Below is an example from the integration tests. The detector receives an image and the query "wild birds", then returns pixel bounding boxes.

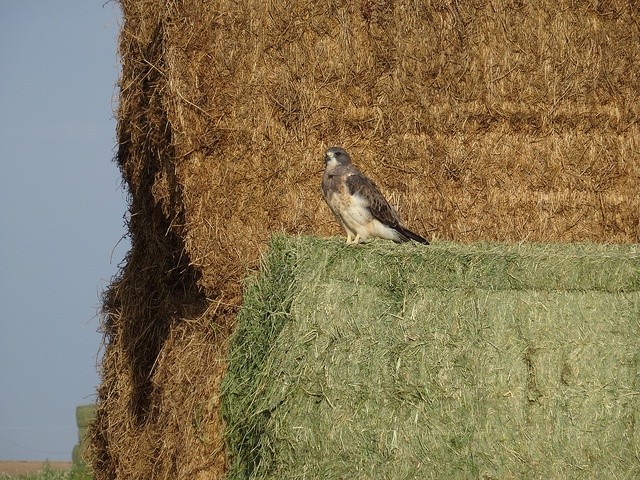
[321,146,429,246]
